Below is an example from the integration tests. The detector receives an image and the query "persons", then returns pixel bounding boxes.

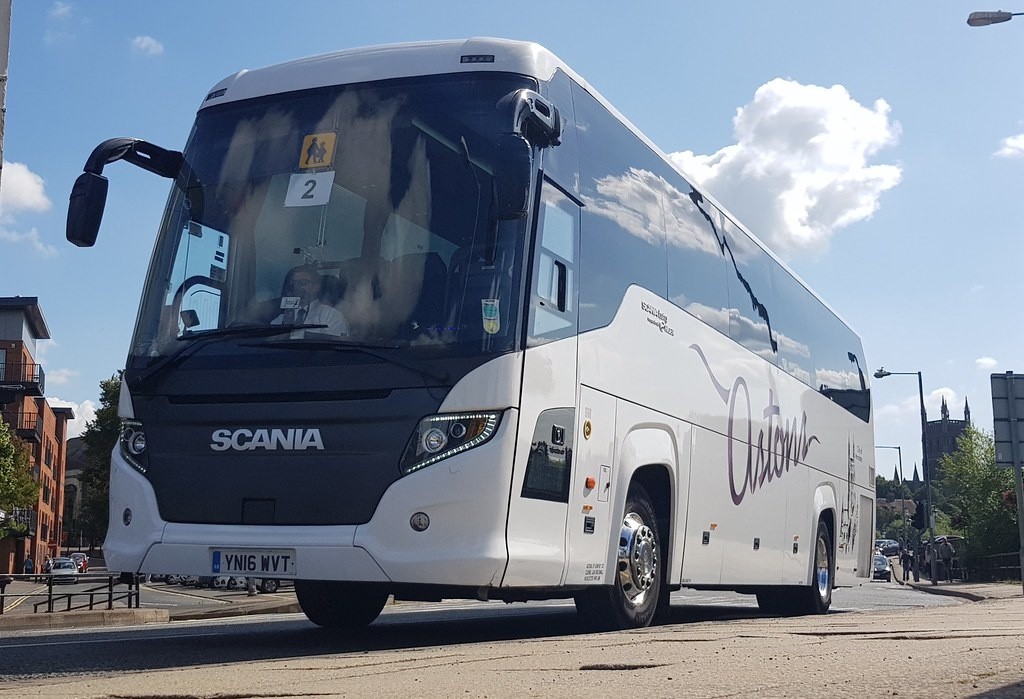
[269,268,349,338]
[43,554,55,581]
[334,257,394,324]
[925,543,937,581]
[82,557,87,573]
[899,549,914,580]
[939,536,955,583]
[24,555,34,580]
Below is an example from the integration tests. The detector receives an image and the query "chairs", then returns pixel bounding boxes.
[319,253,446,303]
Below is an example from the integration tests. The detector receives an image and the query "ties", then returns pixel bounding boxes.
[294,308,306,324]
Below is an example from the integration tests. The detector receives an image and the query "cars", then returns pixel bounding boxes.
[47,559,82,585]
[52,552,91,574]
[875,539,900,556]
[873,554,895,586]
[138,573,294,595]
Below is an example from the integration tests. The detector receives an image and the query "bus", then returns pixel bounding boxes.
[63,35,877,631]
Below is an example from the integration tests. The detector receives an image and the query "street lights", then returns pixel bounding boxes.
[873,364,939,586]
[847,351,866,392]
